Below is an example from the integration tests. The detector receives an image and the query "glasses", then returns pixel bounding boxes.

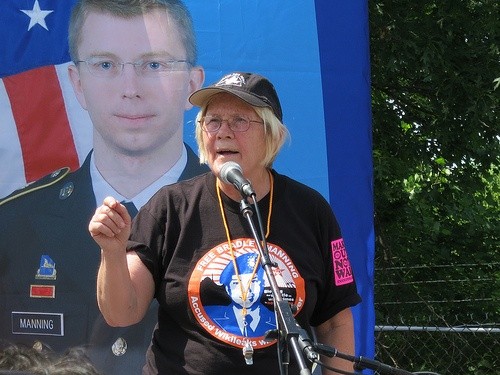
[75,54,193,78]
[196,113,265,133]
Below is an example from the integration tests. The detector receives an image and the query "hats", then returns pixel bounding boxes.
[188,71,283,124]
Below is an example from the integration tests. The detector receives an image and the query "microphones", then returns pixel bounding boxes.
[220,161,256,198]
[293,319,318,363]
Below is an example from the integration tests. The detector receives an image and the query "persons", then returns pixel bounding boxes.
[88,71,363,375]
[0,0,212,375]
[0,337,99,375]
